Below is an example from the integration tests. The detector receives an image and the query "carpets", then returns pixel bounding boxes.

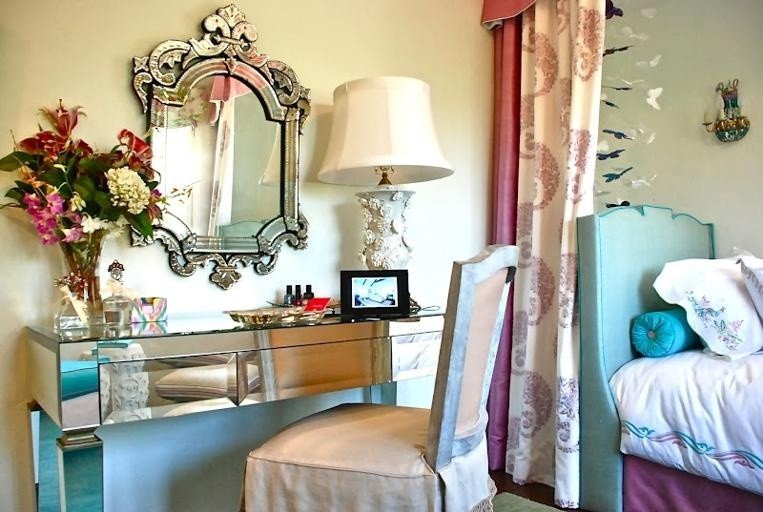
[490,489,567,512]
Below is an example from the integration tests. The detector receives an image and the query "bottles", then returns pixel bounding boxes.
[104,273,133,328]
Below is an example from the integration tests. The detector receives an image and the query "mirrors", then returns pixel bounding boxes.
[133,3,309,290]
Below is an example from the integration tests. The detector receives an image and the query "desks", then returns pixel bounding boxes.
[24,309,445,511]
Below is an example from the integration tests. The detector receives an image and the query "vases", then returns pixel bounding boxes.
[51,230,104,331]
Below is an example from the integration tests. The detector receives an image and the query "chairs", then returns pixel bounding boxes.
[238,242,520,512]
[154,361,262,403]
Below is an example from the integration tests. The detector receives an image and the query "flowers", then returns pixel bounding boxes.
[1,99,193,304]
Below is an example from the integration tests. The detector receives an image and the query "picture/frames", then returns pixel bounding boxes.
[339,270,409,320]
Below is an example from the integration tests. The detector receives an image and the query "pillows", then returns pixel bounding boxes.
[651,247,763,360]
[630,307,697,357]
[737,251,763,321]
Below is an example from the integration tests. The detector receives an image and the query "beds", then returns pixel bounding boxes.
[580,205,763,510]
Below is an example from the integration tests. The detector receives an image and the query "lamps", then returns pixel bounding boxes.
[701,79,750,143]
[318,74,455,309]
